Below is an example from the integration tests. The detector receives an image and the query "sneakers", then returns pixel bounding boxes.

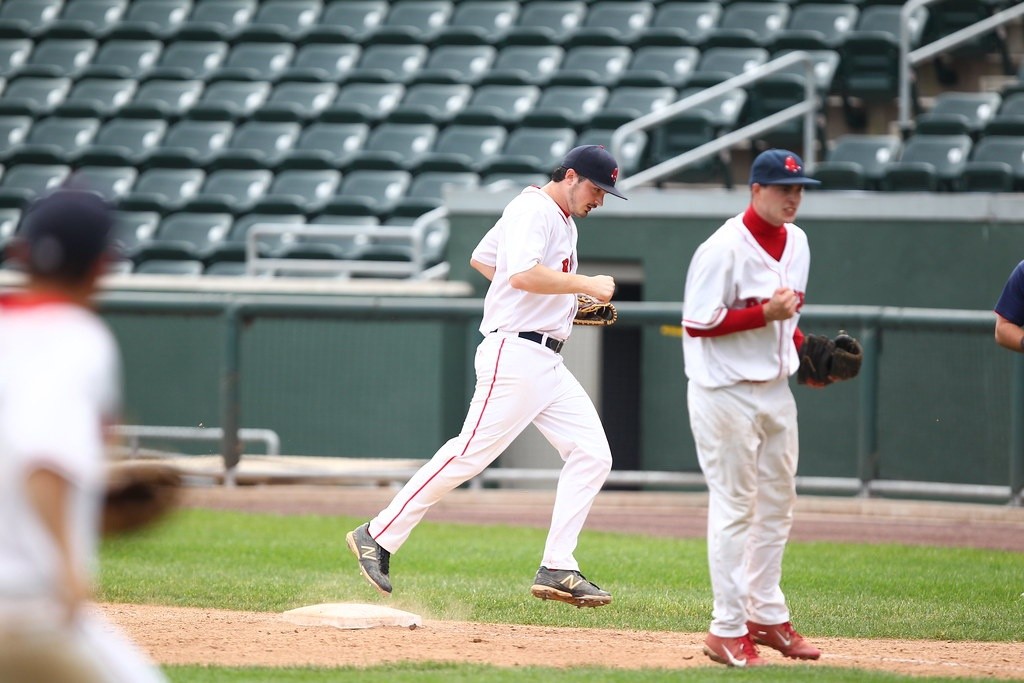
[745,620,821,660]
[345,521,392,593]
[703,632,760,668]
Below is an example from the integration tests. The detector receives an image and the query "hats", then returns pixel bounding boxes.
[749,147,822,187]
[561,144,628,201]
[530,566,611,607]
[0,189,115,282]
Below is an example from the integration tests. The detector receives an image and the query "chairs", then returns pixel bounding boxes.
[0,0,1024,282]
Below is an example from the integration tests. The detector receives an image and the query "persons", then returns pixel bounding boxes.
[0,196,170,683]
[344,146,618,605]
[994,259,1024,352]
[680,149,862,668]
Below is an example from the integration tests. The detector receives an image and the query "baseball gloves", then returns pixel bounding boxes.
[569,292,617,326]
[795,328,864,390]
